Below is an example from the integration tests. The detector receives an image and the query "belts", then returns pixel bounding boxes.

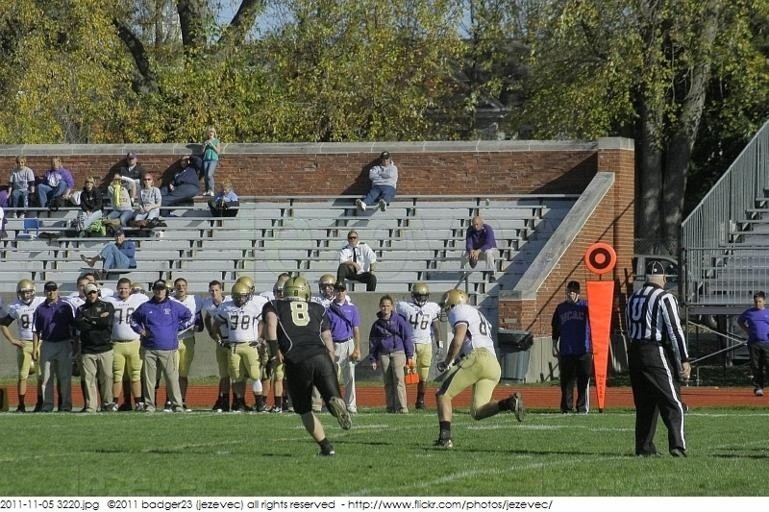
[335,337,353,343]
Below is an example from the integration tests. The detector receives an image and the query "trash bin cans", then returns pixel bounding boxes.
[497,329,532,385]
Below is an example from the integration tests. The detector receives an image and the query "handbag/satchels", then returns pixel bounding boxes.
[84,216,167,237]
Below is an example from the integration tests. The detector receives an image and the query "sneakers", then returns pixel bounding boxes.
[80,254,95,267]
[331,397,352,431]
[669,446,689,458]
[356,198,388,212]
[510,392,525,422]
[317,449,334,456]
[638,453,663,458]
[431,439,453,451]
[16,403,427,415]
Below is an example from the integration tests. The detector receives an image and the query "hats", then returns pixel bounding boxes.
[44,282,57,291]
[127,151,135,157]
[334,280,345,291]
[153,280,167,291]
[646,260,667,275]
[84,283,98,294]
[381,150,389,159]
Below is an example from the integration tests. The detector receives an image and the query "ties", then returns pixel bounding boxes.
[353,248,356,263]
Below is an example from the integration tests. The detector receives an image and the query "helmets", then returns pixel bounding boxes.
[132,283,145,292]
[232,283,251,296]
[438,289,470,307]
[273,278,287,290]
[165,278,175,290]
[16,279,36,296]
[284,276,312,302]
[318,274,336,286]
[567,280,580,292]
[237,276,255,292]
[411,282,431,295]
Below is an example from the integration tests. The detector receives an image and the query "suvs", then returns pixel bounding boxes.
[632,253,718,330]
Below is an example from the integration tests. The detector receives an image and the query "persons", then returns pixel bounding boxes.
[355,151,398,211]
[428,288,526,450]
[0,126,362,456]
[335,230,377,293]
[463,215,500,272]
[737,290,769,396]
[368,295,416,416]
[624,260,691,459]
[550,281,593,414]
[395,283,444,410]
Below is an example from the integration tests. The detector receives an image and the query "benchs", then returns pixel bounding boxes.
[696,188,769,306]
